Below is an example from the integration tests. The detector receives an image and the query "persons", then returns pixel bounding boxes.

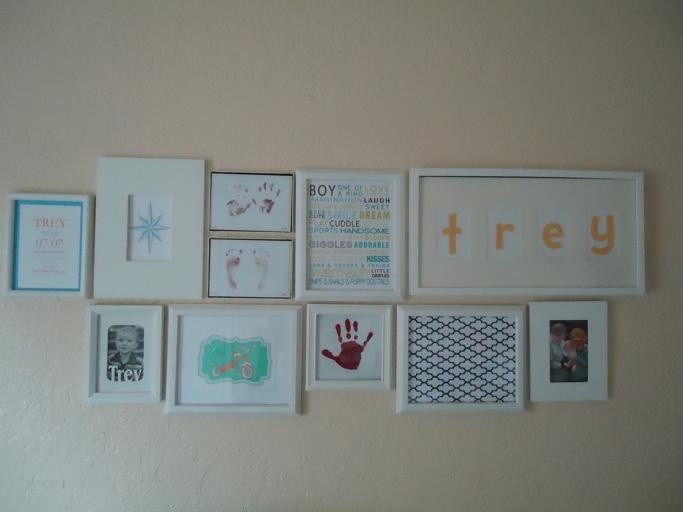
[107,325,143,382]
[549,322,586,383]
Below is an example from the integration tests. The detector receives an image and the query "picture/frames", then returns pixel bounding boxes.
[92,155,205,300]
[396,302,527,413]
[526,300,608,403]
[206,237,293,300]
[207,169,293,234]
[294,168,407,304]
[305,304,394,391]
[81,303,164,404]
[408,166,646,298]
[2,190,94,300]
[164,304,304,418]
